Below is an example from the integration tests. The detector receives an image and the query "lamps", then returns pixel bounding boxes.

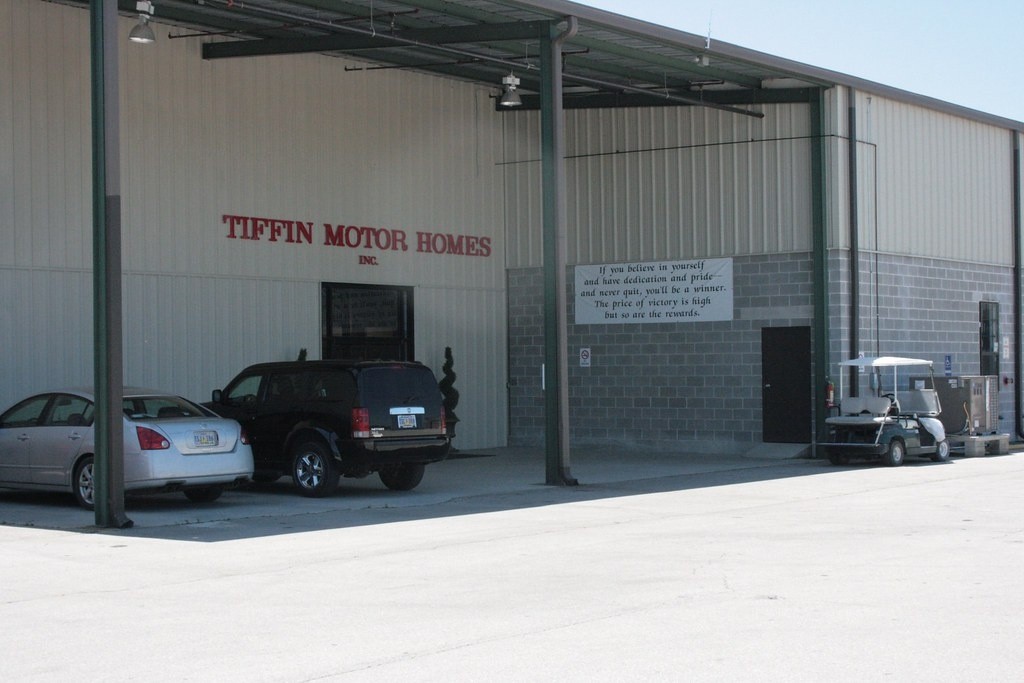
[128,0,156,43]
[500,69,522,107]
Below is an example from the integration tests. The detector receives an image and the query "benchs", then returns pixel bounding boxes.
[825,397,893,424]
[889,398,918,429]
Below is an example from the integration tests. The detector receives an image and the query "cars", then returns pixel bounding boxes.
[0,389,256,509]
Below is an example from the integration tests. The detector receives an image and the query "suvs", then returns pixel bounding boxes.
[158,360,451,501]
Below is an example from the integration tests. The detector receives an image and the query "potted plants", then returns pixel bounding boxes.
[438,346,461,438]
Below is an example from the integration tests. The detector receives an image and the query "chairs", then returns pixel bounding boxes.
[158,406,178,417]
[124,407,134,418]
[68,414,86,426]
